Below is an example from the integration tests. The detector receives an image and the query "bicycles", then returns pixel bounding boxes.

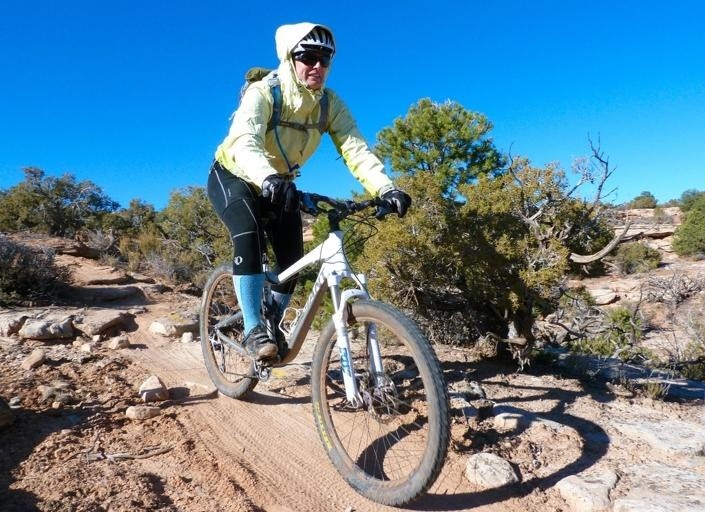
[197,187,452,506]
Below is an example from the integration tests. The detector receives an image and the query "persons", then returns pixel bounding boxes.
[206,20,412,360]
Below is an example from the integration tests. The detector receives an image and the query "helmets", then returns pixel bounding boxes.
[291,28,335,58]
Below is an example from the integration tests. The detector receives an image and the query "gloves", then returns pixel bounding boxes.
[374,188,411,219]
[263,172,301,214]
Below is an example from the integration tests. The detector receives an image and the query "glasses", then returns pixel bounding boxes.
[293,53,329,68]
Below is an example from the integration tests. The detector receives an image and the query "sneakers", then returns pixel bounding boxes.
[241,324,280,361]
